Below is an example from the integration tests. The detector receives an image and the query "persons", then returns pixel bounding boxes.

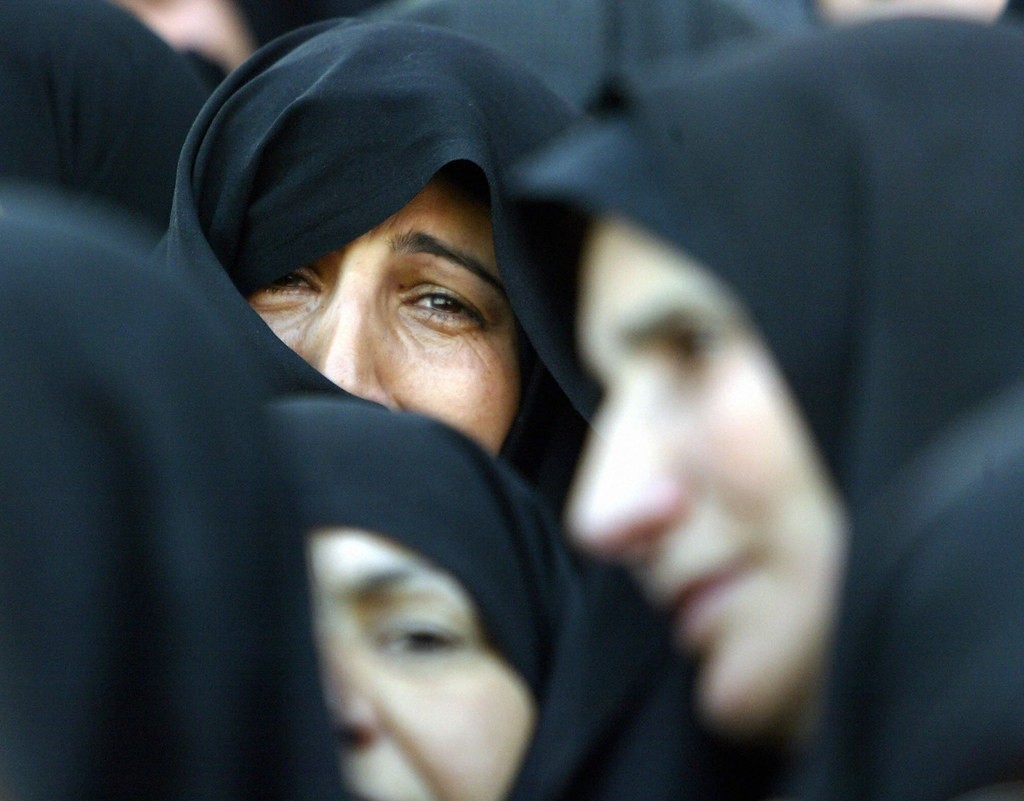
[277,400,565,801]
[167,20,591,516]
[505,18,1024,801]
